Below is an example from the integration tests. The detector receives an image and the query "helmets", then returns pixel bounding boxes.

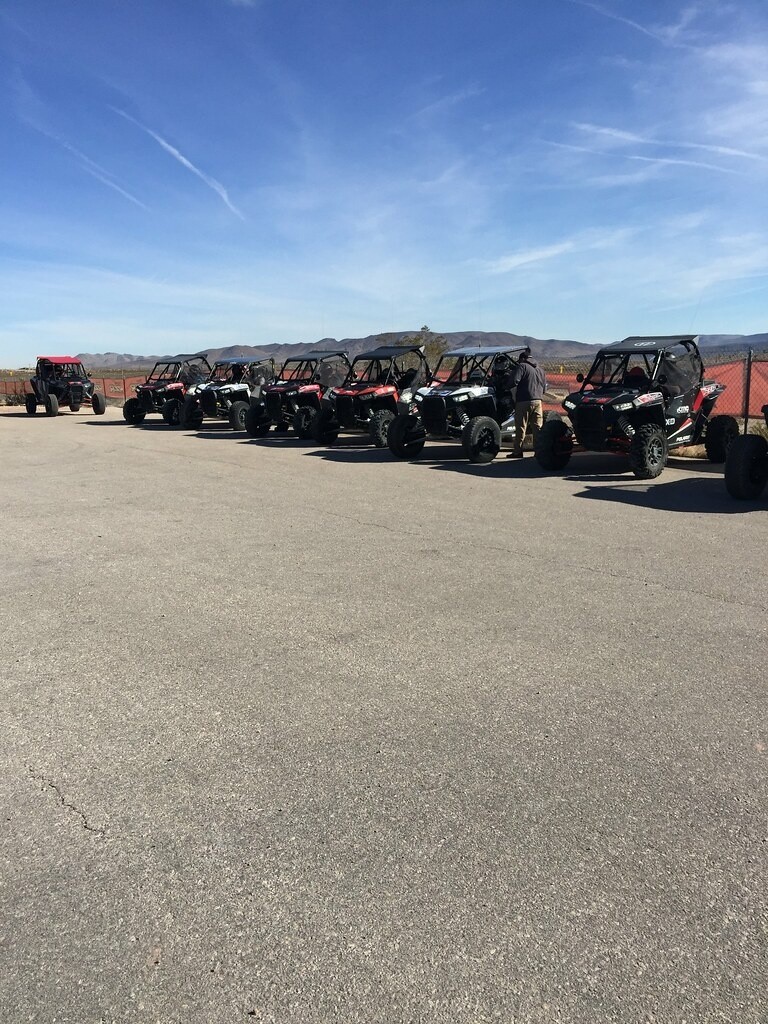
[652,352,676,362]
[493,356,509,370]
[469,369,482,380]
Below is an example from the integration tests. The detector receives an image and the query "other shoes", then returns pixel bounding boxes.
[507,453,523,458]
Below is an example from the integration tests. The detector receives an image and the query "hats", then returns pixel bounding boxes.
[515,351,530,362]
[628,367,644,375]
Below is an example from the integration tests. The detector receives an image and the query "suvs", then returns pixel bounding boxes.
[25,355,106,417]
[532,334,742,480]
[177,355,280,431]
[243,348,362,438]
[723,401,768,502]
[386,344,562,465]
[122,351,220,426]
[307,343,441,447]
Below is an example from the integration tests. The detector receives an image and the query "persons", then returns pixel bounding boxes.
[490,356,516,418]
[55,364,68,378]
[231,365,249,386]
[318,363,342,389]
[374,366,416,391]
[625,367,648,388]
[245,364,264,386]
[186,365,204,384]
[505,352,546,459]
[653,352,690,394]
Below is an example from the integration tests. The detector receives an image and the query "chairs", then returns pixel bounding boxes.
[190,364,205,382]
[396,368,418,389]
[624,367,650,385]
[171,370,188,383]
[376,368,398,382]
[231,367,248,383]
[470,369,482,382]
